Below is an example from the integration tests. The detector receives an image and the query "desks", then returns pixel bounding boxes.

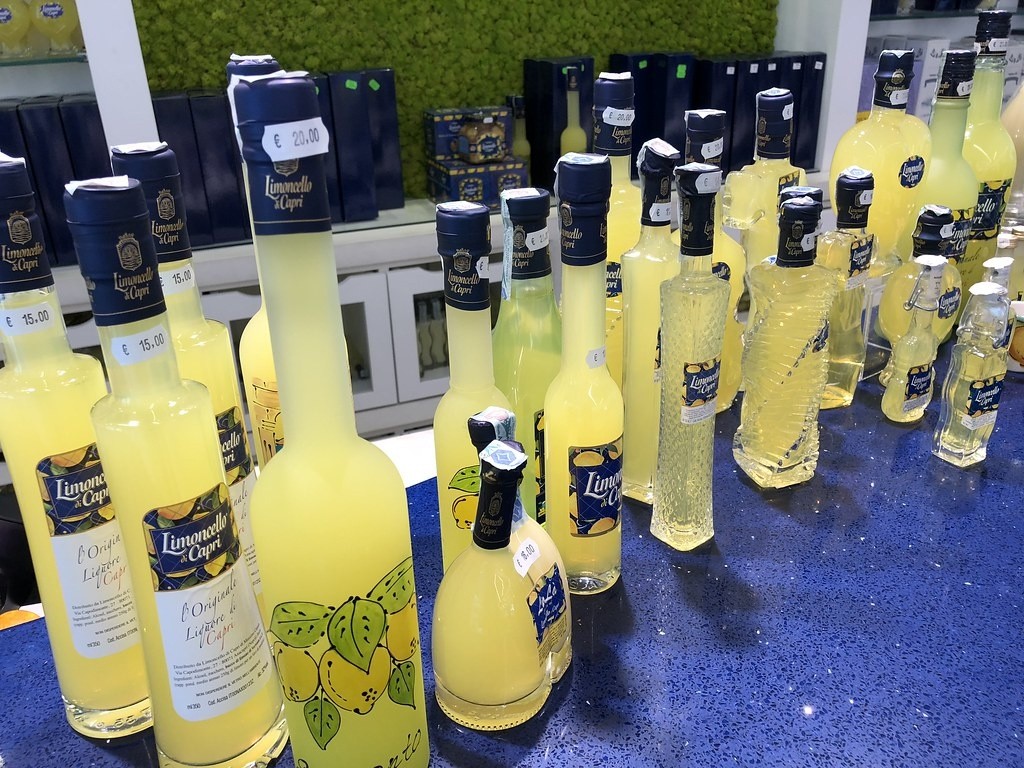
[0,304,1024,768]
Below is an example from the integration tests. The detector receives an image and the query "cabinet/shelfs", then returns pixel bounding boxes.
[773,0,1024,210]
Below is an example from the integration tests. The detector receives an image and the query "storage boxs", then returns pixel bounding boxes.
[362,66,405,210]
[188,89,247,245]
[524,56,580,197]
[609,52,653,180]
[858,33,1024,126]
[470,106,514,156]
[308,72,343,224]
[578,55,595,153]
[691,51,826,180]
[440,164,489,207]
[423,157,457,204]
[152,92,214,248]
[0,95,58,268]
[421,107,475,162]
[320,70,380,222]
[489,159,528,212]
[18,95,78,267]
[648,52,698,181]
[59,93,113,180]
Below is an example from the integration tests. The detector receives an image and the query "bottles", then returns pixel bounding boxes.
[62,176,288,768]
[232,73,431,768]
[0,157,153,739]
[226,49,290,471]
[432,0,1024,732]
[98,141,259,602]
